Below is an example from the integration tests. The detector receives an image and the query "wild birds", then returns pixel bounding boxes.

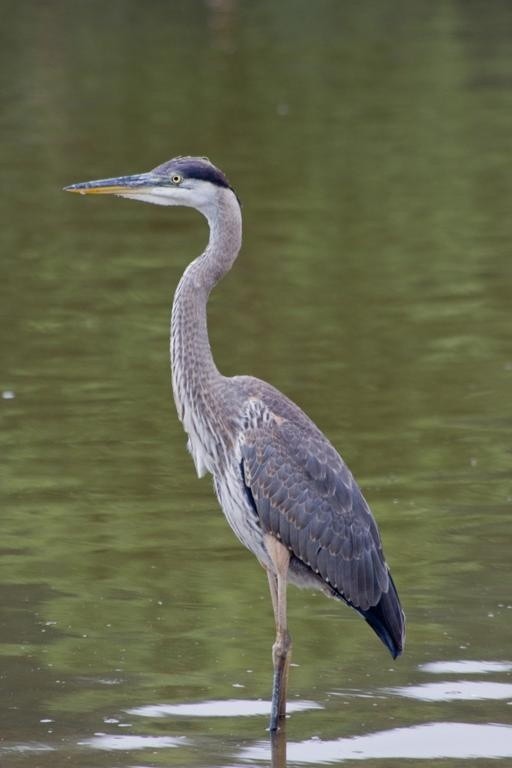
[60,153,406,736]
